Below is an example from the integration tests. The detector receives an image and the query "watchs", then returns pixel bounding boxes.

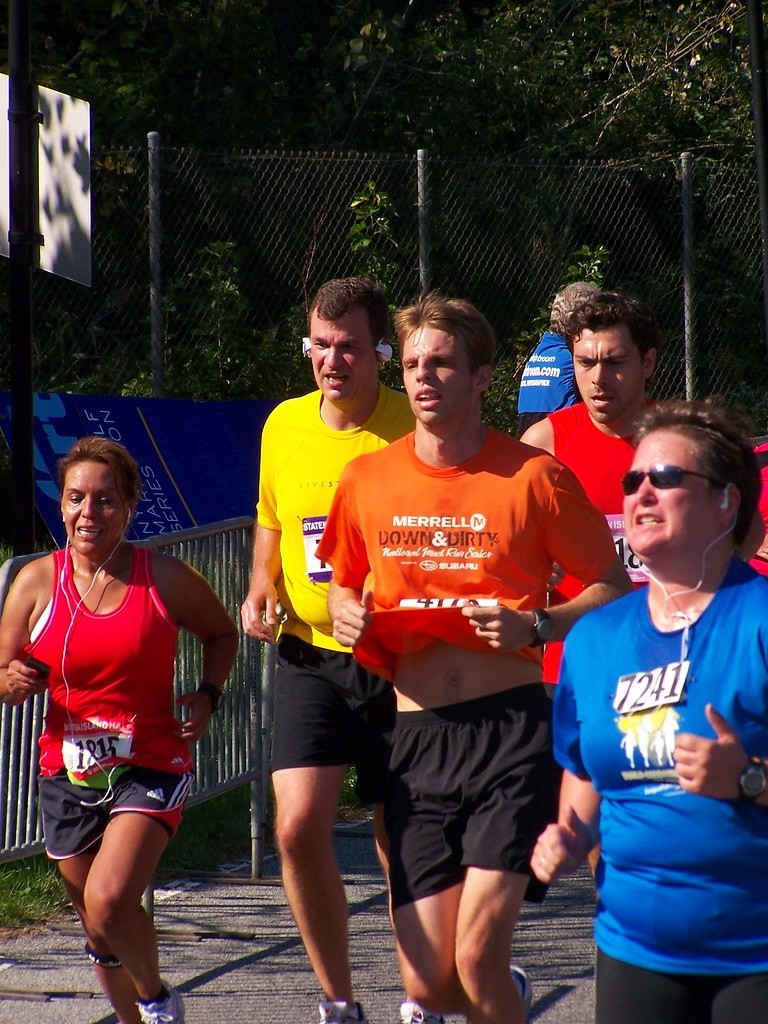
[530,608,556,649]
[736,752,767,806]
[197,681,223,715]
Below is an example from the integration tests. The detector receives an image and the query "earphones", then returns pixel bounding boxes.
[720,488,729,510]
[376,337,392,362]
[63,516,65,522]
[128,508,131,523]
[302,337,311,358]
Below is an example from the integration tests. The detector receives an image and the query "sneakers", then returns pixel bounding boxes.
[318,1001,368,1024]
[400,1002,444,1024]
[509,965,530,1023]
[136,979,184,1024]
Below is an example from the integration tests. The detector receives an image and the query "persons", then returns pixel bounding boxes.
[515,282,599,435]
[0,435,241,1024]
[315,291,631,1024]
[526,398,768,1024]
[520,294,668,874]
[242,282,534,1023]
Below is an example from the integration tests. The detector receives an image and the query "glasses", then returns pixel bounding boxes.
[620,466,725,495]
